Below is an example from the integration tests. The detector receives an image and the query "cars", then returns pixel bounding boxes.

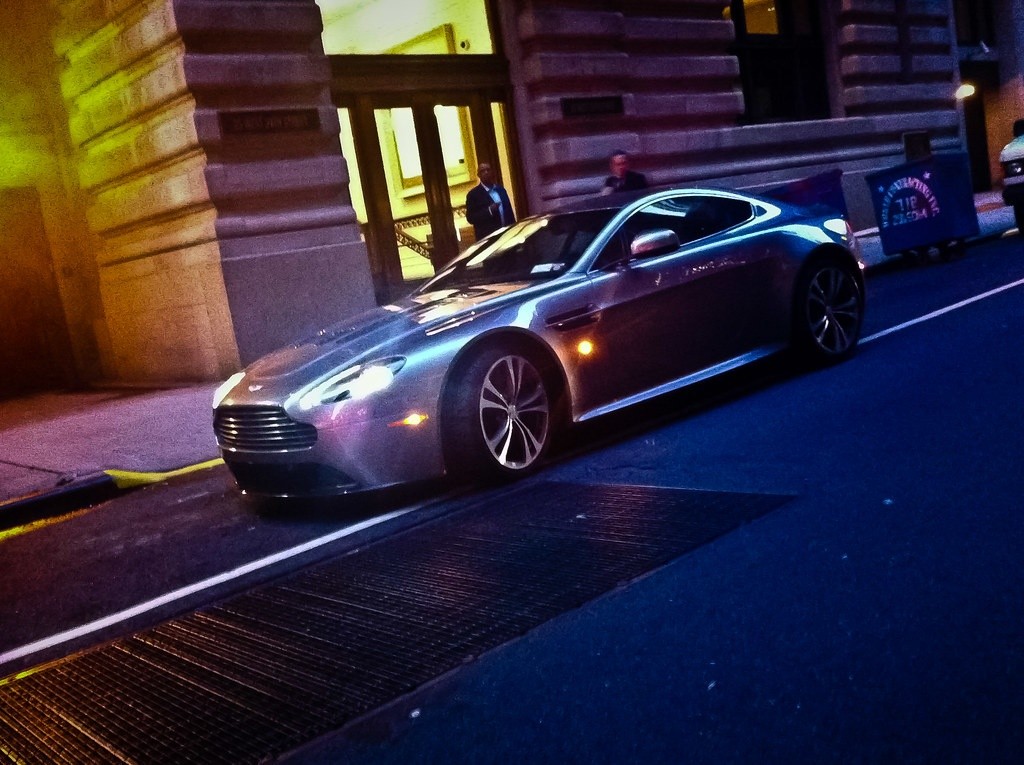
[208,185,869,505]
[998,133,1024,236]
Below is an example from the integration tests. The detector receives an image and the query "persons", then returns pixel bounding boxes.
[999,117,1024,238]
[602,151,646,195]
[466,163,516,242]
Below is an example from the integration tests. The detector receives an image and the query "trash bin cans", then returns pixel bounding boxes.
[863,150,982,256]
[757,168,850,221]
